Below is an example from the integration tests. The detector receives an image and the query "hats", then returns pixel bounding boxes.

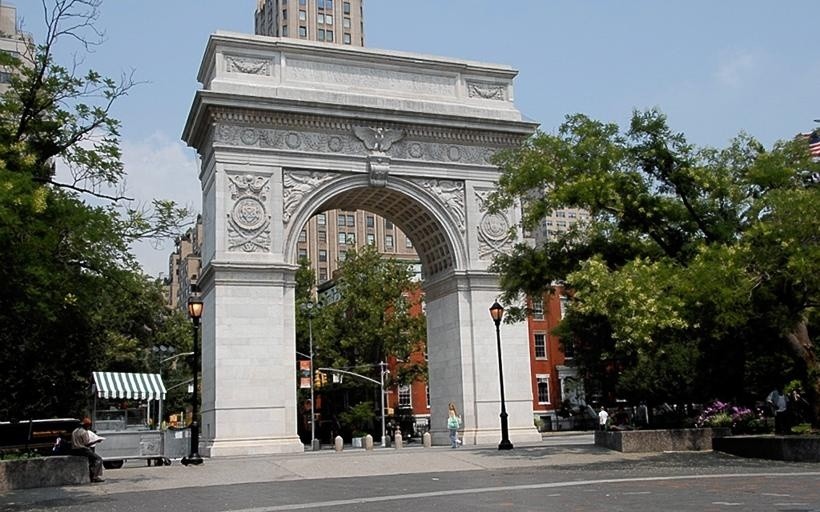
[78,417,93,426]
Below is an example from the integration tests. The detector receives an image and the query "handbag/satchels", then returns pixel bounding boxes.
[446,410,464,430]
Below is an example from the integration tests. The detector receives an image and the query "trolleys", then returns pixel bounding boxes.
[86,371,173,467]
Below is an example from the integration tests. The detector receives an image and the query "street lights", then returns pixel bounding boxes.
[185,293,204,465]
[490,297,515,450]
[152,344,175,374]
[305,300,323,451]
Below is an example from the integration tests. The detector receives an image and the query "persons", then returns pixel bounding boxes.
[447,403,461,448]
[765,383,789,430]
[598,406,608,432]
[70,417,105,483]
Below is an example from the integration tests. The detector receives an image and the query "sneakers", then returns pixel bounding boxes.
[91,476,105,482]
